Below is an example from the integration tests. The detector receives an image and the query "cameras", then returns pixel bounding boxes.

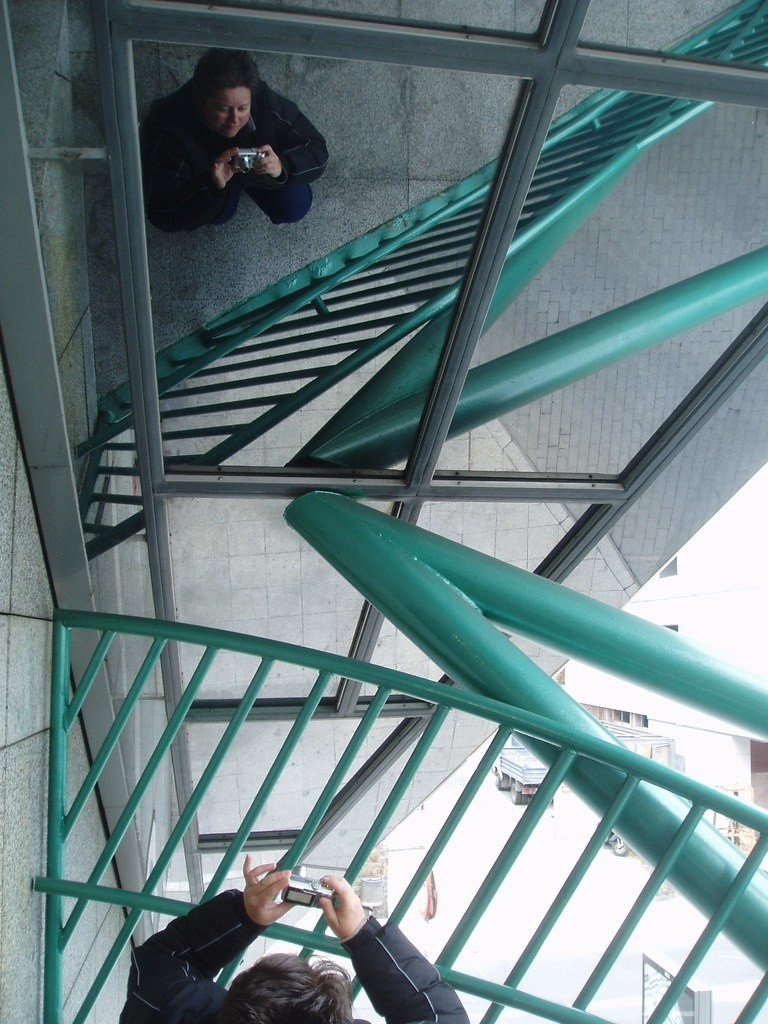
[231,147,266,174]
[281,875,334,909]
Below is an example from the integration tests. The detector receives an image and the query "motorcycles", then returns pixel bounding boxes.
[605,831,629,857]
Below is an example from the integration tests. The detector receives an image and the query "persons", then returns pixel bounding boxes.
[118,853,467,1024]
[141,47,328,233]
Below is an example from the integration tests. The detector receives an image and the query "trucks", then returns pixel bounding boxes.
[492,747,554,806]
